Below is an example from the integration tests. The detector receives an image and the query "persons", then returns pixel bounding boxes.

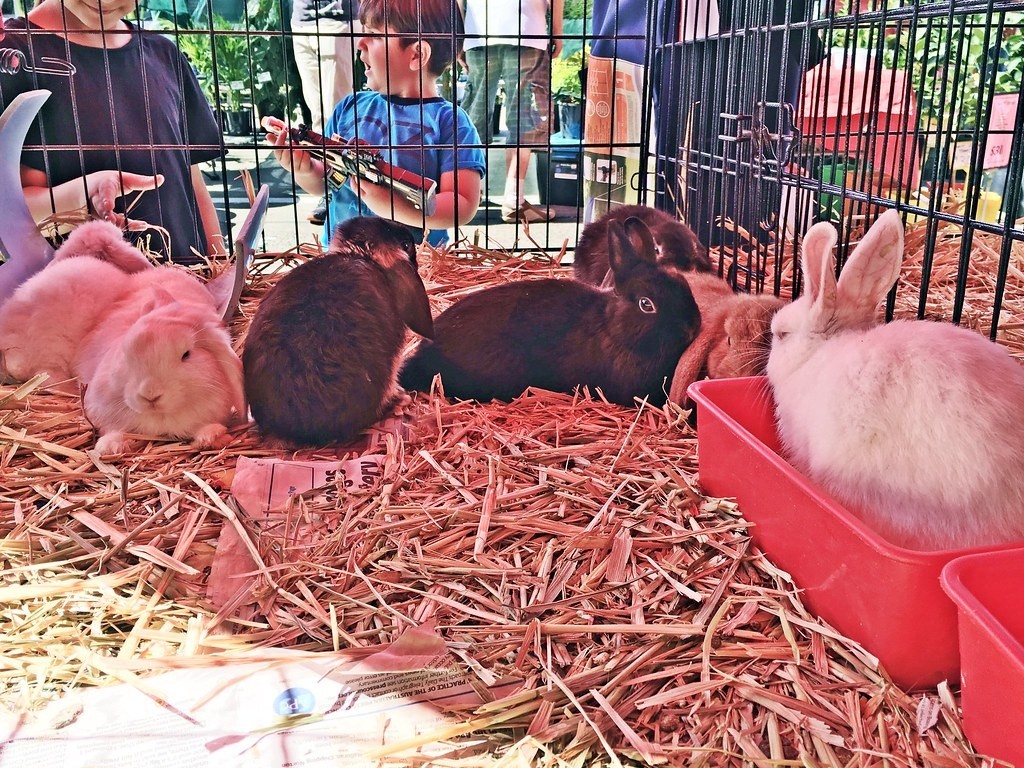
[645,0,828,249]
[456,0,562,223]
[0,0,231,270]
[265,0,487,255]
[290,0,363,223]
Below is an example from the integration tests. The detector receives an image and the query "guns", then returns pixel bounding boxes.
[259,115,439,218]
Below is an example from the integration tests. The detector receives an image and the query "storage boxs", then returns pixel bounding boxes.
[537,131,584,208]
[687,376,1024,691]
[940,546,1024,768]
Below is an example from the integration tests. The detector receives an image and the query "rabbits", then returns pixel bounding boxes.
[394,215,703,409]
[0,217,155,389]
[71,263,248,457]
[667,266,786,408]
[241,216,437,447]
[571,203,714,290]
[764,205,1024,552]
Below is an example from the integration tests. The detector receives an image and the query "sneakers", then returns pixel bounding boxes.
[306,194,332,222]
[502,200,556,222]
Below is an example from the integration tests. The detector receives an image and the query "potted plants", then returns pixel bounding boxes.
[174,11,287,135]
[558,69,583,140]
